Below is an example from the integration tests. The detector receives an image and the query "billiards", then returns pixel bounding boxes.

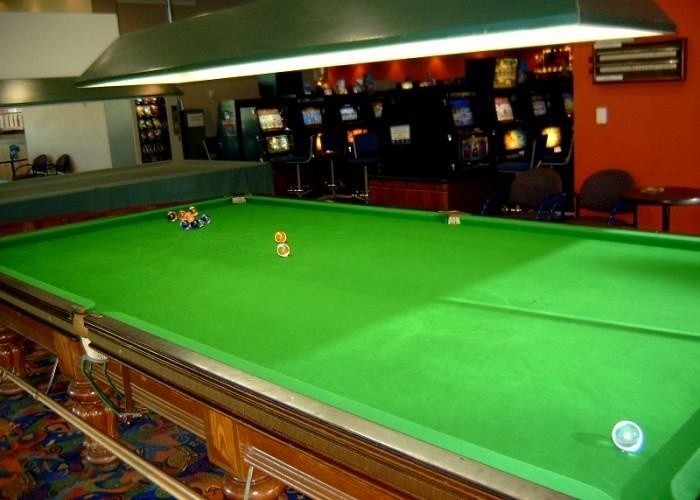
[167,211,177,222]
[178,206,211,231]
[275,232,286,243]
[612,420,644,454]
[277,245,291,259]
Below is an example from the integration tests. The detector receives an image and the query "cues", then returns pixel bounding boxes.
[252,446,354,498]
[432,296,699,340]
[246,453,329,498]
[248,448,343,498]
[243,457,317,498]
[1,368,202,500]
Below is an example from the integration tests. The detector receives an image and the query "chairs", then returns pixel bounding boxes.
[500,140,537,176]
[39,154,69,177]
[578,168,638,231]
[541,127,574,169]
[13,155,46,180]
[202,137,226,160]
[284,132,378,203]
[479,168,566,223]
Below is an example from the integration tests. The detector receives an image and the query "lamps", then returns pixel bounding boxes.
[592,36,686,85]
[74,1,678,91]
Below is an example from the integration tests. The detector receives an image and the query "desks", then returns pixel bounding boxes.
[370,172,485,211]
[625,186,700,232]
[2,161,275,238]
[1,195,700,500]
[0,157,27,182]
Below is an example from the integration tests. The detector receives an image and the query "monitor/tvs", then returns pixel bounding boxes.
[256,103,411,154]
[448,93,574,161]
